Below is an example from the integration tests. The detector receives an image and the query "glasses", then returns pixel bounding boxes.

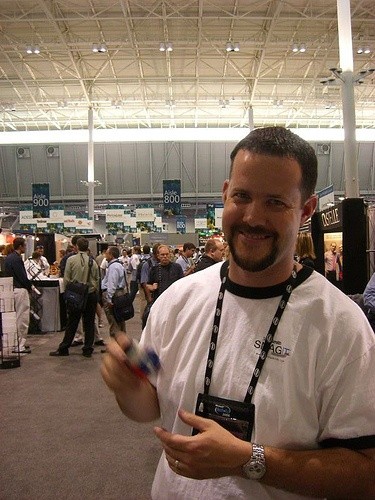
[36,245,44,250]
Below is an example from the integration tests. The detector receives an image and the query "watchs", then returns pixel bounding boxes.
[240,443,266,481]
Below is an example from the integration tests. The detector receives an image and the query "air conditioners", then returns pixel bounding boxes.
[45,145,59,157]
[316,144,330,155]
[16,146,31,159]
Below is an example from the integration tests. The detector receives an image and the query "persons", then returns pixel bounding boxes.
[363,272,375,310]
[118,242,184,305]
[24,245,50,280]
[324,242,343,282]
[49,235,128,358]
[4,237,36,354]
[0,244,15,257]
[295,232,316,268]
[168,238,225,276]
[100,126,374,500]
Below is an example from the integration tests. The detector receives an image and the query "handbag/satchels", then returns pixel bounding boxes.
[110,293,134,324]
[64,279,89,309]
[142,304,150,330]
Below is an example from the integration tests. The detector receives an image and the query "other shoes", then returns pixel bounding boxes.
[70,341,83,346]
[49,349,70,356]
[82,351,91,357]
[24,345,30,349]
[20,350,31,354]
[30,329,49,334]
[94,340,104,346]
[98,318,104,328]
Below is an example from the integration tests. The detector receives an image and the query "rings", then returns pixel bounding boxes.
[175,460,179,467]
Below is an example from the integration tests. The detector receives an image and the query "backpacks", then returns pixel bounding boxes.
[137,258,149,280]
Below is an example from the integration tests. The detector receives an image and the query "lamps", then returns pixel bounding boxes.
[90,42,107,53]
[160,42,174,52]
[26,44,41,55]
[355,46,371,54]
[293,43,306,52]
[226,42,241,53]
